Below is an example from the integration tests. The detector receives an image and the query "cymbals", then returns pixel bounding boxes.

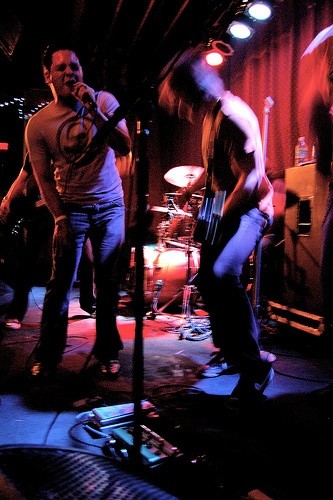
[163,165,207,188]
[164,192,204,199]
[150,206,194,217]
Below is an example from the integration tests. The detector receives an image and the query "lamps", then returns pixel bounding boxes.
[204,0,285,66]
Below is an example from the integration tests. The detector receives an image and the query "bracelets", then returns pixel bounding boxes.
[189,185,195,190]
[54,215,68,224]
[3,196,8,199]
[90,108,99,113]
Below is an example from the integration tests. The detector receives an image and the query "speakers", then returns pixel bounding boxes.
[280,161,331,328]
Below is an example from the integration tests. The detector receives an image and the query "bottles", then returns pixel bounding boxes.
[294,136,308,166]
[312,138,318,160]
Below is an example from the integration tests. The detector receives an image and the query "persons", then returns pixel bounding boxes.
[160,58,274,400]
[0,44,136,385]
[309,72,333,334]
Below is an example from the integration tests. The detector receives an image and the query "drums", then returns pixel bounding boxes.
[136,241,201,309]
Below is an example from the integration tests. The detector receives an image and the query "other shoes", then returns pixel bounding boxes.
[29,354,51,380]
[2,317,22,330]
[95,357,122,379]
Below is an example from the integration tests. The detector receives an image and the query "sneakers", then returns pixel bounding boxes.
[198,356,237,378]
[227,362,275,411]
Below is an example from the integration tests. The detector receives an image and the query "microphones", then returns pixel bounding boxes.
[69,82,96,107]
